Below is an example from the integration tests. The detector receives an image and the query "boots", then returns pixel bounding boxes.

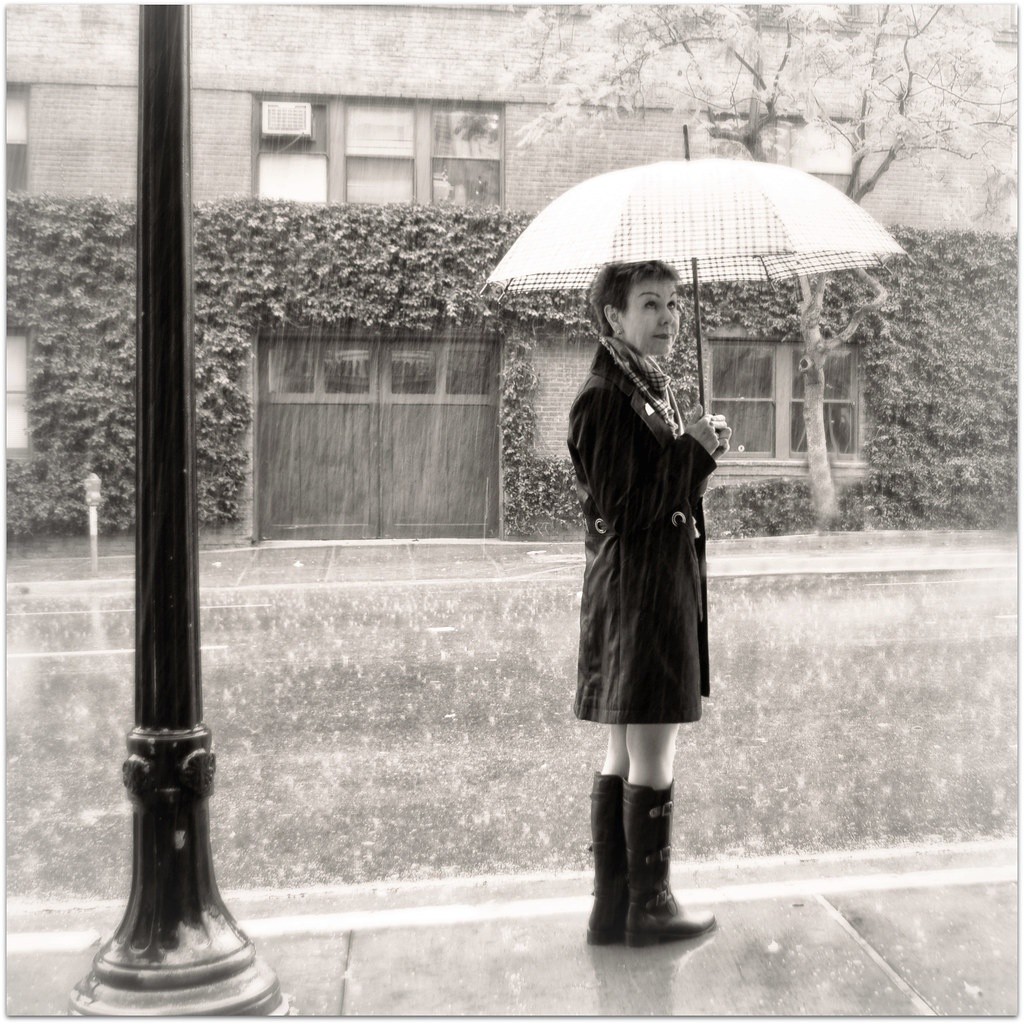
[623,778,717,947]
[587,771,628,944]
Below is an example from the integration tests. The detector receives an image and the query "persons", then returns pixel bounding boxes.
[565,261,732,947]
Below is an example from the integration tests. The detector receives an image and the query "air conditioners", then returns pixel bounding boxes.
[262,99,311,141]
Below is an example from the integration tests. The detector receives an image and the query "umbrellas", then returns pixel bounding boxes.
[477,126,911,414]
